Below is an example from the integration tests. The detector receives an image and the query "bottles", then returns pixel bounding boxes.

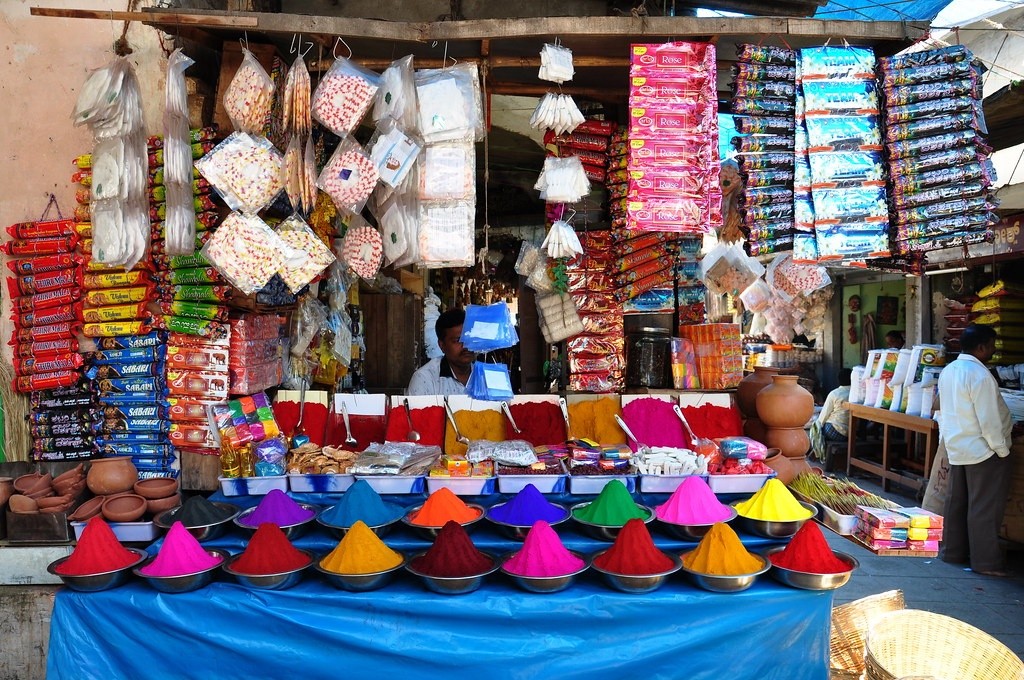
[625,327,672,388]
[737,367,822,501]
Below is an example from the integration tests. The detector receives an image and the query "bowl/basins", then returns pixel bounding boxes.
[0,457,861,591]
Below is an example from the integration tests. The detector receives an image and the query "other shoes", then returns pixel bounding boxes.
[980,565,1019,577]
[943,553,971,563]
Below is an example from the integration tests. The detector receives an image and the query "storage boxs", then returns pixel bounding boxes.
[216,456,779,494]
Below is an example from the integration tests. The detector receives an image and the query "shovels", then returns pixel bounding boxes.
[295,378,307,436]
[673,404,722,452]
[403,397,421,441]
[613,414,651,453]
[342,401,358,448]
[443,396,474,447]
[500,400,522,434]
[559,397,582,441]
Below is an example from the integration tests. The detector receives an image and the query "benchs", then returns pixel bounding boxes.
[824,434,905,471]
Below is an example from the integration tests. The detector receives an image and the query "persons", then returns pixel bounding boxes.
[407,307,474,397]
[938,325,1014,577]
[809,330,907,462]
